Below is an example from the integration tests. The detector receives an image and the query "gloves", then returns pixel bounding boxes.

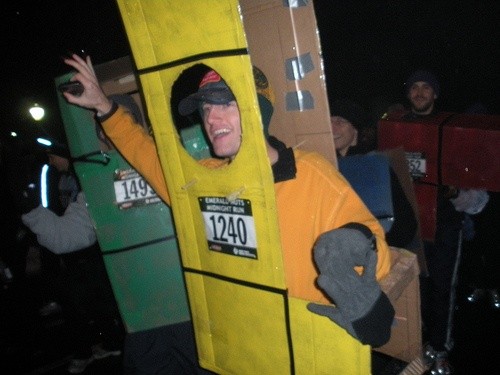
[305,222,397,347]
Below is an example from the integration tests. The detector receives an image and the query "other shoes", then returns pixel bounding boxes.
[66,343,121,373]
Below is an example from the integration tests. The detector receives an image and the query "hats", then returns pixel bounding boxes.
[402,71,440,91]
[329,95,361,130]
[178,61,274,118]
